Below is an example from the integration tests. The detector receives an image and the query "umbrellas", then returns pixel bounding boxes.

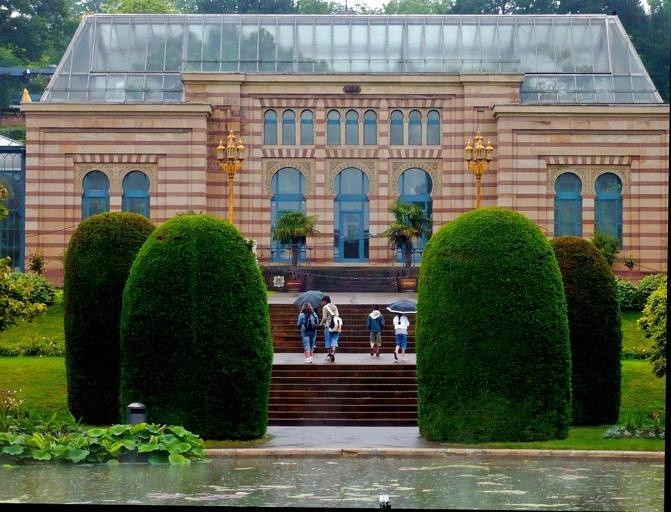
[386,300,418,313]
[293,290,325,309]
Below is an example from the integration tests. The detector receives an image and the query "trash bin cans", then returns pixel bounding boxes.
[128,402,147,424]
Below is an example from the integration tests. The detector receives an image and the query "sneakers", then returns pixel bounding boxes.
[370,348,379,356]
[305,357,313,364]
[325,353,336,364]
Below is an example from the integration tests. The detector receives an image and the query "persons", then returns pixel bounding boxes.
[297,296,343,364]
[367,305,410,360]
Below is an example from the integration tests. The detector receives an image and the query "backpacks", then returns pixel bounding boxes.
[323,305,343,334]
[303,310,319,330]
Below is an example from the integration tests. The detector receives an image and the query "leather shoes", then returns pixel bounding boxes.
[393,351,407,361]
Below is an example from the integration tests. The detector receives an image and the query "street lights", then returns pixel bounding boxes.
[462,128,496,212]
[213,127,248,226]
